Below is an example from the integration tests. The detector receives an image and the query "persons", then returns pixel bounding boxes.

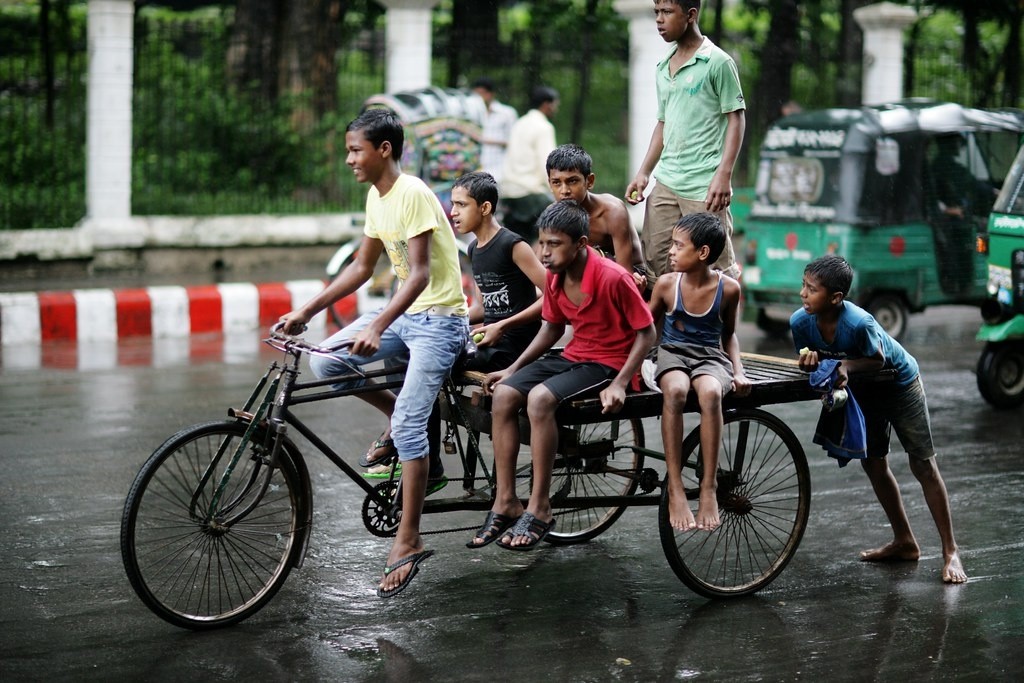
[539,144,659,363]
[789,255,968,583]
[279,109,470,599]
[648,212,751,533]
[500,89,557,215]
[925,132,1023,237]
[469,78,519,186]
[624,1,746,334]
[362,171,548,497]
[463,200,658,552]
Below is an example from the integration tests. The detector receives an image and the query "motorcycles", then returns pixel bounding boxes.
[743,98,1024,346]
[971,145,1024,417]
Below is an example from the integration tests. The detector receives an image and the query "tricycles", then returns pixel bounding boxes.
[324,216,470,330]
[121,321,827,632]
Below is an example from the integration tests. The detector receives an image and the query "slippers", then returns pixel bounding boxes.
[361,463,403,479]
[465,510,521,549]
[358,431,397,468]
[377,548,435,597]
[421,473,451,498]
[495,511,556,551]
